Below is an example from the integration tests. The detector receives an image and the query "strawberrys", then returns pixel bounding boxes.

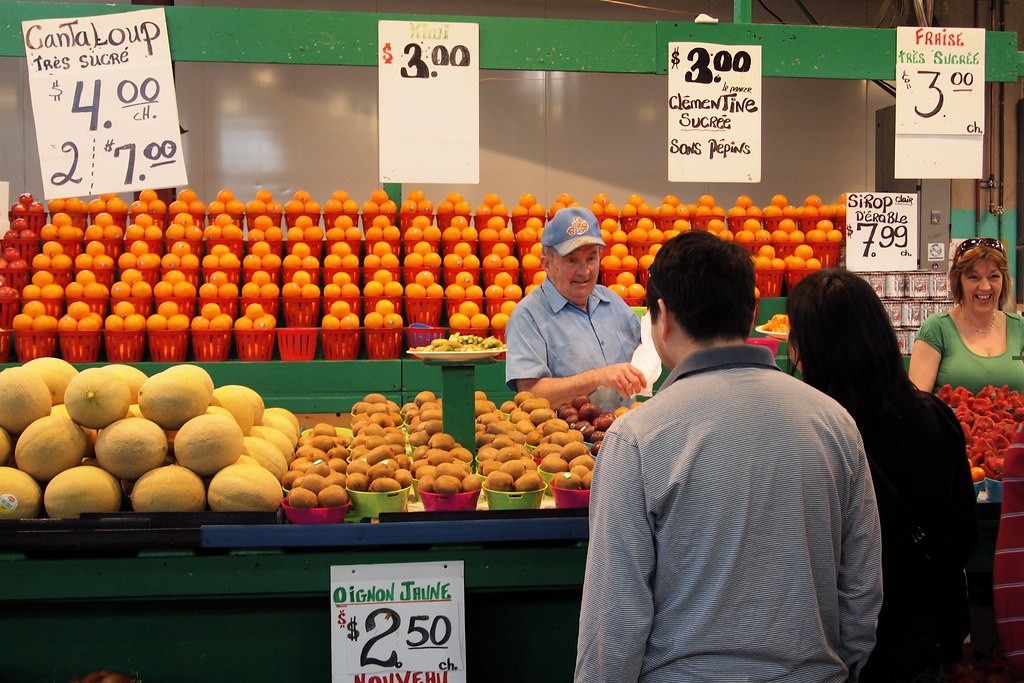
[937,384,1024,481]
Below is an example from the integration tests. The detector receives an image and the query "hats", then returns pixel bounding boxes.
[541,206,607,256]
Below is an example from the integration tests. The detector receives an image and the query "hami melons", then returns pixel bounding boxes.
[1,356,298,519]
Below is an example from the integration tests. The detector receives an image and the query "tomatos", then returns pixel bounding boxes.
[1,192,44,300]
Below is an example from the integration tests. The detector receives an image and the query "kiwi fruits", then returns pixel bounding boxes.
[281,391,596,507]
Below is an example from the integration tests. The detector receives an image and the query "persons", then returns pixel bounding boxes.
[505,206,648,413]
[573,229,884,683]
[908,238,1024,394]
[785,267,981,683]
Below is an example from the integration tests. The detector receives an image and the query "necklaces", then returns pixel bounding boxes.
[959,304,996,334]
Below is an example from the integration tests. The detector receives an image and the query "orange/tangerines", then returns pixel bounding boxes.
[10,189,864,329]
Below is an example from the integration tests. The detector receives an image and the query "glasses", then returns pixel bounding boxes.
[958,238,1005,256]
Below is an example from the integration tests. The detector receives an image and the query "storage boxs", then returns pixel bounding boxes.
[278,410,1024,523]
[0,215,848,358]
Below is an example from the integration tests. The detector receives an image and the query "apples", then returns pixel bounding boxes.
[556,394,615,455]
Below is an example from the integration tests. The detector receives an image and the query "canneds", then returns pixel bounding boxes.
[854,271,955,354]
